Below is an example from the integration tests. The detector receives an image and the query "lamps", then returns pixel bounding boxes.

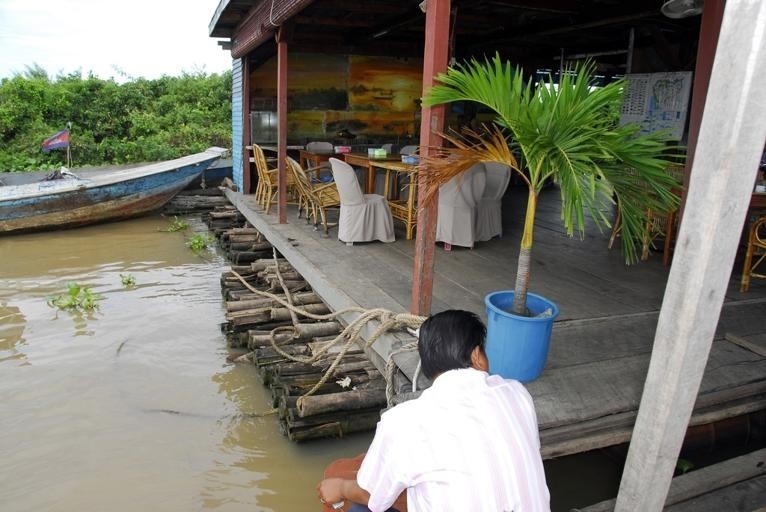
[662,0,704,18]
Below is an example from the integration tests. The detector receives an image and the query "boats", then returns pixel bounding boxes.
[0,147,230,239]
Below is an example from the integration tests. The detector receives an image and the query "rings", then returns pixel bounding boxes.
[319,497,326,503]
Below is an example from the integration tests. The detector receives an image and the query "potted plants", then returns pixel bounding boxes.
[424,52,689,385]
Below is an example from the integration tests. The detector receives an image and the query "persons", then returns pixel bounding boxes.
[315,306,555,512]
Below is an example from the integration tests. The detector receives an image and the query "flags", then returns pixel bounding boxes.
[39,130,69,152]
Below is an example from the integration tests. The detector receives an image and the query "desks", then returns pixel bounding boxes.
[342,151,408,194]
[368,158,419,241]
[299,148,346,180]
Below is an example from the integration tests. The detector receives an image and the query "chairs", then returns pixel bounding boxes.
[285,155,342,238]
[513,144,764,292]
[434,151,512,252]
[380,143,396,155]
[400,145,419,157]
[251,143,295,215]
[328,157,396,247]
[306,144,334,183]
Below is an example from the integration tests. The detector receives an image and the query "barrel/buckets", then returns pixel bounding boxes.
[485,289,559,384]
